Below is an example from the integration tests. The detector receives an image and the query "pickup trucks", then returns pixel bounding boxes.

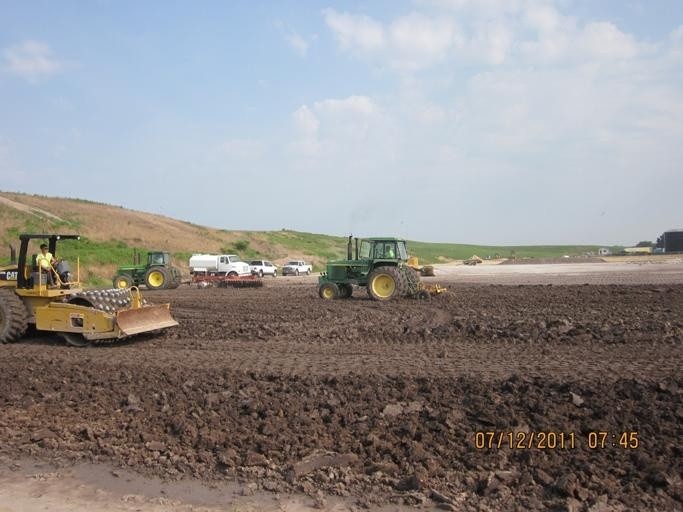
[280,259,313,276]
[249,260,278,277]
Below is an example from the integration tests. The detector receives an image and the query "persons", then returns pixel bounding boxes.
[379,245,395,258]
[34,243,72,289]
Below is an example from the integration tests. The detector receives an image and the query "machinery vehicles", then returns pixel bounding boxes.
[111,246,182,292]
[315,235,422,302]
[0,234,179,346]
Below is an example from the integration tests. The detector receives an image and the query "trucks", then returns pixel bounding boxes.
[188,252,251,279]
